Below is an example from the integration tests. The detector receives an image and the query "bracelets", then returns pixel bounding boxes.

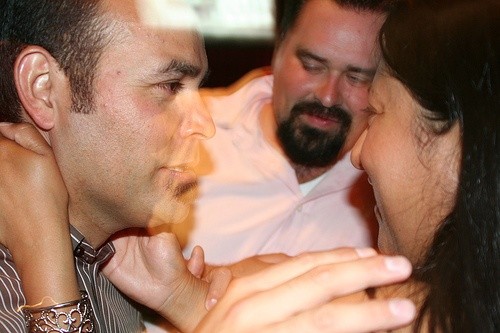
[23,289,95,332]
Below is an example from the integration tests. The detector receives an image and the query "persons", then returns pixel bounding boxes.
[0,0,500,332]
[0,0,417,332]
[140,0,394,332]
